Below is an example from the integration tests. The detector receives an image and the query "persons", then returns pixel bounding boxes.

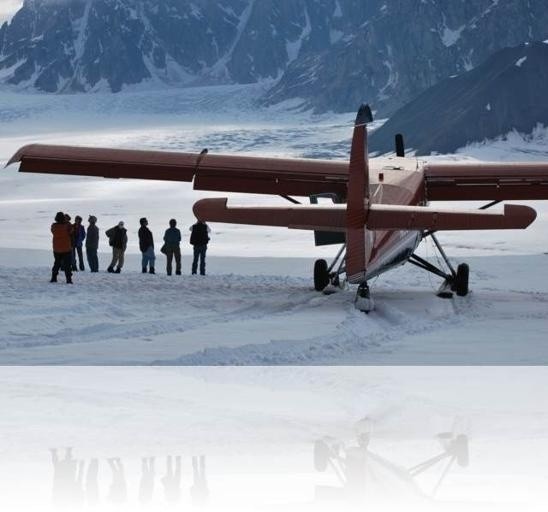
[86,215,99,272]
[49,212,86,284]
[138,218,156,273]
[106,221,128,273]
[163,219,181,275]
[189,220,211,275]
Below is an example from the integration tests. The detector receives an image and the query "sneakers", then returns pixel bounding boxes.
[142,267,156,274]
[59,263,85,271]
[108,265,121,274]
[166,270,182,276]
[192,270,206,276]
[89,268,99,272]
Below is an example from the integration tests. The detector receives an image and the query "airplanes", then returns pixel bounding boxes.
[5,103,548,314]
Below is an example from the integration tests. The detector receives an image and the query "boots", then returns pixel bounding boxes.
[64,272,74,284]
[48,270,59,282]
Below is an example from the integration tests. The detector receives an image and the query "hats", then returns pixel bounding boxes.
[89,214,97,222]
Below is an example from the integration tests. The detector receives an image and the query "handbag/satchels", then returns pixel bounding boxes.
[160,244,167,256]
